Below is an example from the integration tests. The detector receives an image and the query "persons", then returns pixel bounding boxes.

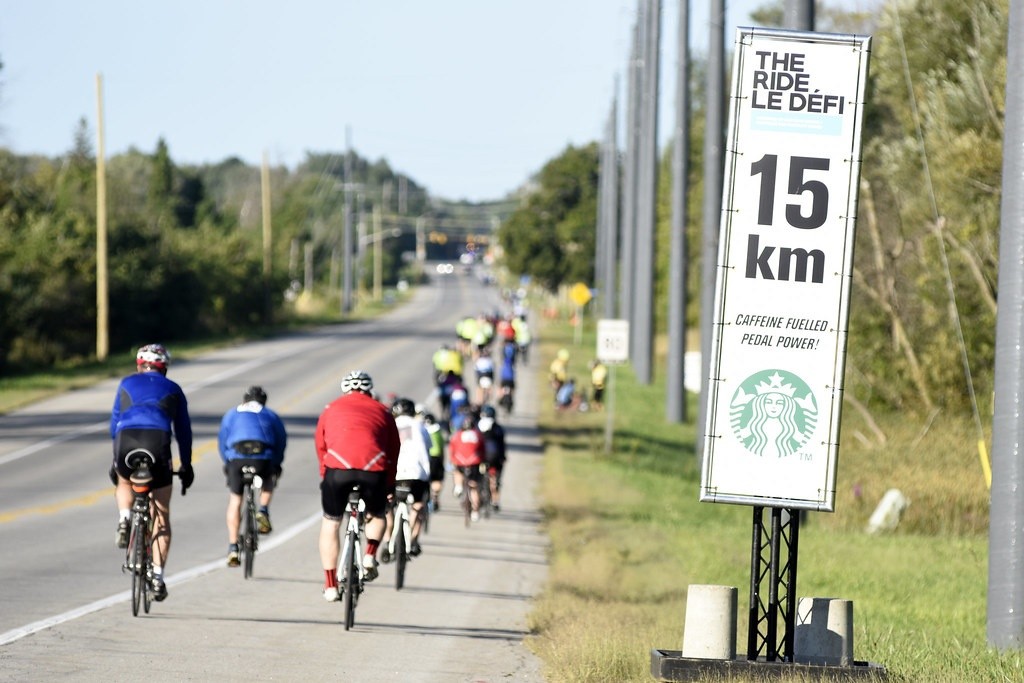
[383,392,449,512]
[551,352,606,412]
[432,311,532,521]
[316,371,401,602]
[109,345,194,602]
[379,399,433,564]
[217,385,287,567]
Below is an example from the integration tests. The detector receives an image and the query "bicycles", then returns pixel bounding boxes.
[109,456,187,617]
[234,463,271,579]
[330,479,379,631]
[457,462,500,526]
[389,476,421,590]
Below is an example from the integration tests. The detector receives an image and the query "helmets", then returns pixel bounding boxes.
[341,372,373,394]
[481,407,495,416]
[138,345,169,373]
[245,386,267,406]
[461,418,476,430]
[392,399,416,415]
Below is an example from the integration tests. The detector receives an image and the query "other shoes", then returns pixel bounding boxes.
[227,551,241,570]
[324,587,341,602]
[152,581,165,597]
[256,510,272,533]
[363,555,379,579]
[116,523,130,549]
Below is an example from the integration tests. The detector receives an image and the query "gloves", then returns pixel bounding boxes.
[179,468,195,491]
[109,467,117,484]
